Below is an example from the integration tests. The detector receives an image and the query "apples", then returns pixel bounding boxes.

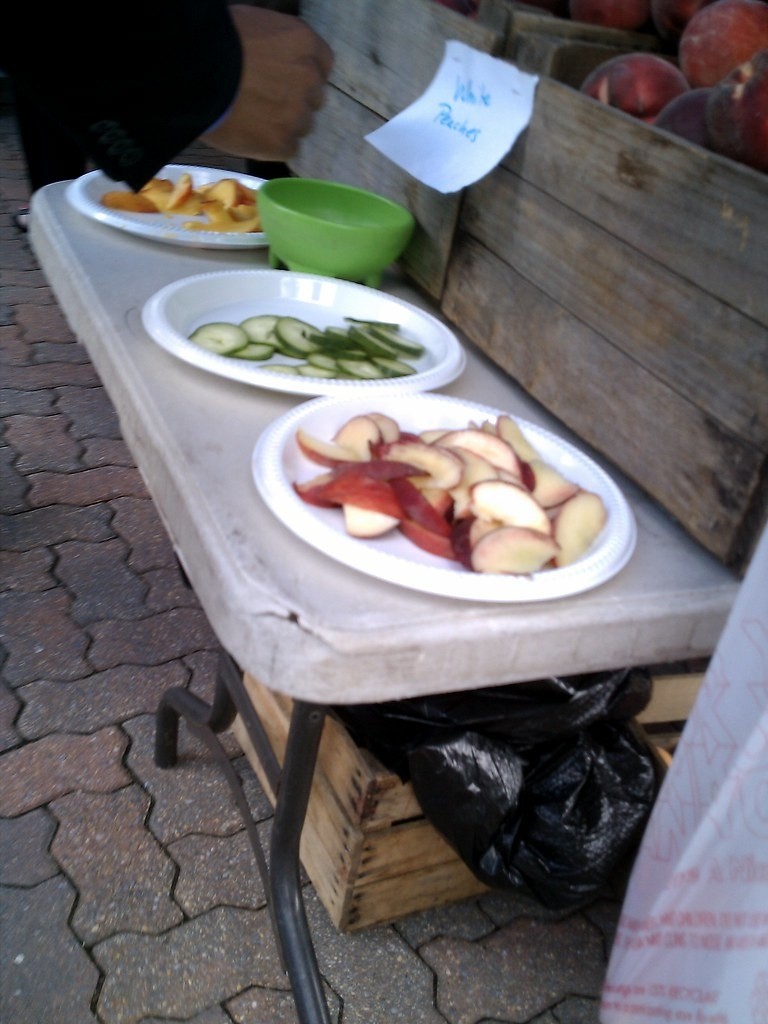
[101,174,268,234]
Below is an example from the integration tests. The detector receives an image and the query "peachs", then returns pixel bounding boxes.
[292,414,607,576]
[420,0,768,177]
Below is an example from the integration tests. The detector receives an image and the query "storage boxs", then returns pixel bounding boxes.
[286,0,768,562]
[233,673,701,934]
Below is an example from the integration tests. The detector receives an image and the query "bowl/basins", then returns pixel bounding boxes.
[256,178,414,292]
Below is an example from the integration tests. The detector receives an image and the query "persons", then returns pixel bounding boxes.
[0,0,248,229]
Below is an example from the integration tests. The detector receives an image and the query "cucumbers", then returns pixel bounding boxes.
[185,314,424,378]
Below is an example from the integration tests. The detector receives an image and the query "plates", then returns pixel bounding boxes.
[141,270,467,396]
[250,395,638,604]
[65,165,269,249]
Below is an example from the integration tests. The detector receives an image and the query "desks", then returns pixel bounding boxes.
[23,179,746,1024]
